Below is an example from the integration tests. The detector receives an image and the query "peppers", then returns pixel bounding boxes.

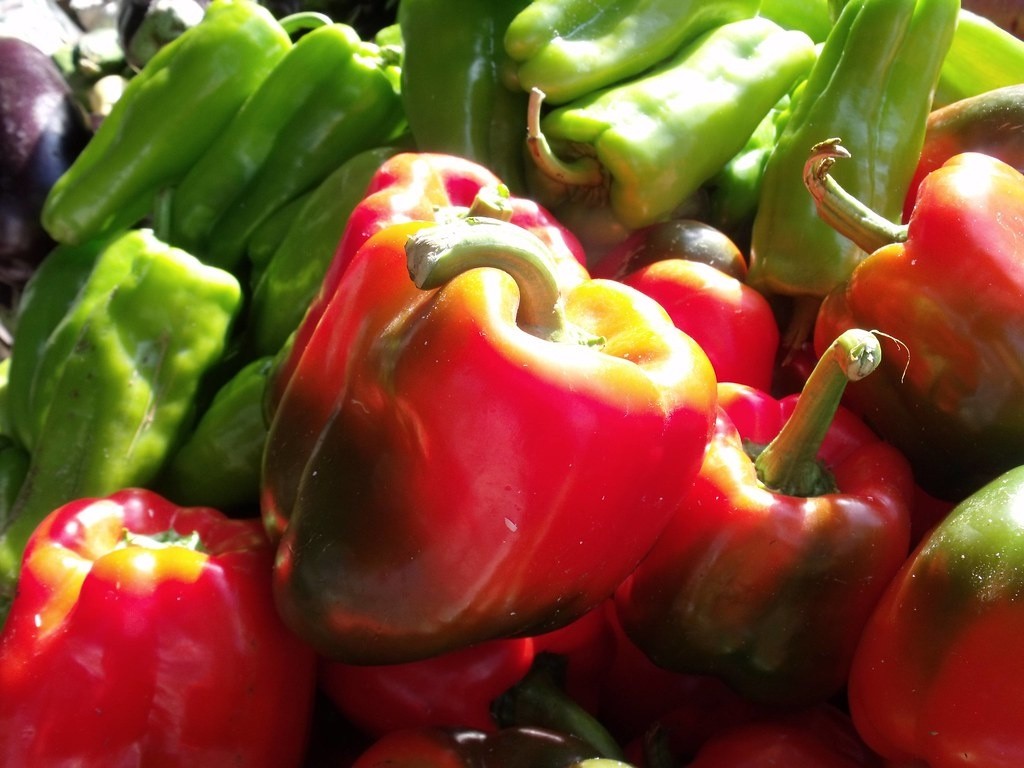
[0,0,1024,768]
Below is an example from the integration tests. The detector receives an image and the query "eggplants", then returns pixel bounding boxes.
[0,34,99,308]
